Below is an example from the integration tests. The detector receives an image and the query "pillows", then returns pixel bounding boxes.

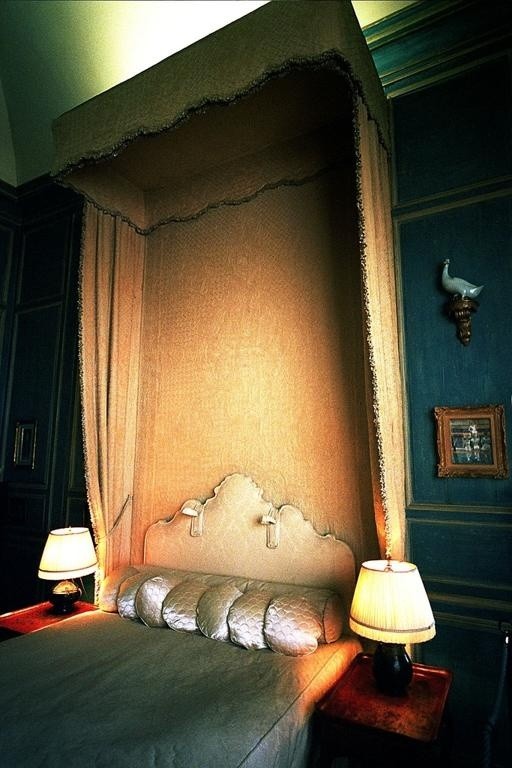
[99,565,345,657]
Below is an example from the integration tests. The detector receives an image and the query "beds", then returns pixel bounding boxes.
[0,0,415,767]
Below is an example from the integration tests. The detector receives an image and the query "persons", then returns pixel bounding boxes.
[462,422,491,461]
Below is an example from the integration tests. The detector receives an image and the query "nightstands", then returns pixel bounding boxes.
[0,601,99,635]
[317,652,454,768]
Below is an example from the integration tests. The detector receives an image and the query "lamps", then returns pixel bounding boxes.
[348,559,437,698]
[38,527,99,613]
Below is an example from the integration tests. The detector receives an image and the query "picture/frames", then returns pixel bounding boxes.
[433,404,512,480]
[12,422,37,470]
